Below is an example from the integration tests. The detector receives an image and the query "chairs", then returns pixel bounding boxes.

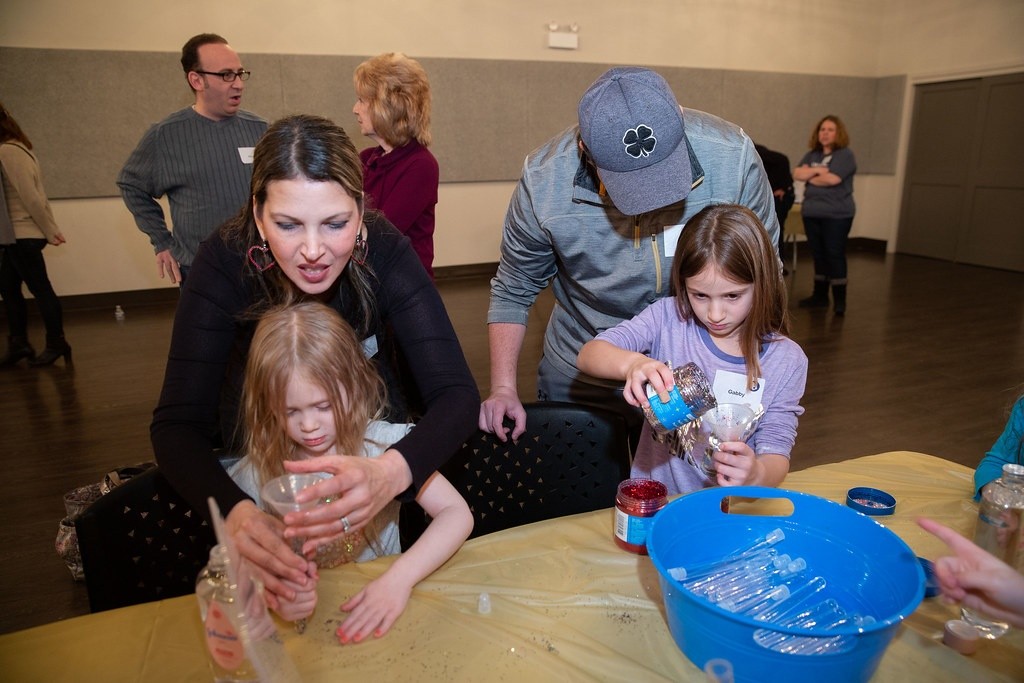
[397,402,644,553]
[76,465,219,610]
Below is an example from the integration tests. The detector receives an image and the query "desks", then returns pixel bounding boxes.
[0,447,1024,683]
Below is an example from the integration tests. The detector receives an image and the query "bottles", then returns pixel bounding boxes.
[196,545,302,683]
[614,479,667,555]
[640,362,716,435]
[961,464,1024,638]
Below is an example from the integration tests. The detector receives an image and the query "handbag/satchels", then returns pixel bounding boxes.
[55,464,158,580]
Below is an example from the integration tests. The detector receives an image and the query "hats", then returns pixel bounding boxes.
[579,67,693,215]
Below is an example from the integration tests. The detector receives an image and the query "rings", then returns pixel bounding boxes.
[340,517,349,531]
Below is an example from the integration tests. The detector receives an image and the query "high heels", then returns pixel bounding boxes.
[0,334,35,368]
[27,331,73,366]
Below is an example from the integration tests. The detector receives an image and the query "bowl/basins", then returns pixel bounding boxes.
[647,486,925,683]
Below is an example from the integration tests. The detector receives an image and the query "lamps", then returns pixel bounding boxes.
[549,19,580,49]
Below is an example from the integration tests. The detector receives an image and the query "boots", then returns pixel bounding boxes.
[797,274,830,307]
[832,278,848,315]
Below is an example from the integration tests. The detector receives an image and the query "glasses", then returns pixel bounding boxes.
[198,70,250,82]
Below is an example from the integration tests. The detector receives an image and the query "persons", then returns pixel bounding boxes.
[972,389,1024,542]
[915,517,1024,629]
[220,301,474,645]
[478,65,784,458]
[117,33,269,290]
[577,203,809,496]
[0,103,73,369]
[352,52,440,281]
[792,115,858,313]
[754,145,796,275]
[149,114,482,608]
